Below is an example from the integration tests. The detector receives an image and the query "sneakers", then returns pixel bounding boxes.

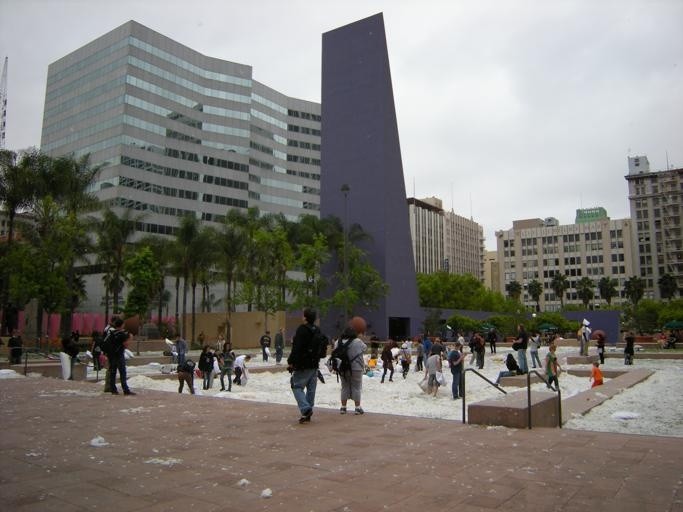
[339,407,346,414]
[299,408,313,424]
[355,408,363,415]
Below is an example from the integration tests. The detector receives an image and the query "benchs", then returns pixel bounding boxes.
[616,343,683,349]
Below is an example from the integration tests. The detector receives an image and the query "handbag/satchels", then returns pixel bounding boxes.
[406,359,410,363]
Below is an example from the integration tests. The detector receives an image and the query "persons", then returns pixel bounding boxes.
[368,331,485,398]
[495,353,520,384]
[62,329,103,372]
[655,329,681,349]
[8,328,24,365]
[512,323,528,375]
[577,317,592,356]
[595,333,605,364]
[259,329,272,362]
[286,307,327,424]
[528,332,543,368]
[587,362,603,390]
[334,326,367,415]
[274,328,285,364]
[488,326,499,352]
[105,316,137,396]
[167,329,252,396]
[545,346,561,392]
[620,329,635,364]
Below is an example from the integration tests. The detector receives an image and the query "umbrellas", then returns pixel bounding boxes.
[662,319,683,329]
[592,330,605,338]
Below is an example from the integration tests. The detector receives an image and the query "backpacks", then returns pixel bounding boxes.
[330,337,354,383]
[103,330,121,353]
[304,325,329,360]
